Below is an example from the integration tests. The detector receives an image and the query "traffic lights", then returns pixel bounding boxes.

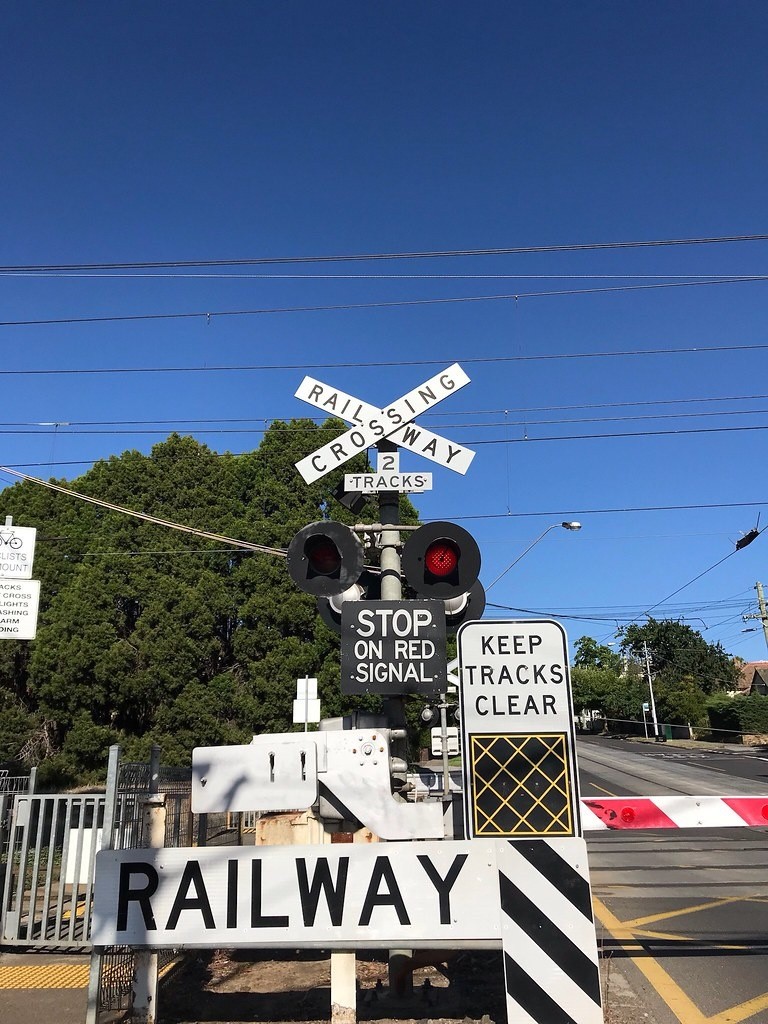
[285,521,363,597]
[401,523,481,602]
